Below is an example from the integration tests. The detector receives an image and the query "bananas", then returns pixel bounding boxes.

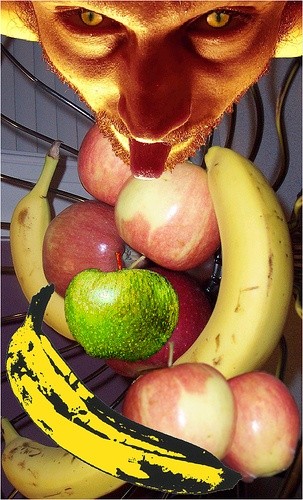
[1,415,126,496]
[172,146,294,378]
[6,284,243,494]
[10,141,76,342]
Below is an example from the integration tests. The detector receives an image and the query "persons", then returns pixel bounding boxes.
[2,1,303,181]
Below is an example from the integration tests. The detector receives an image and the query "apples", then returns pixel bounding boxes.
[64,268,180,360]
[42,198,127,297]
[77,123,134,205]
[221,371,299,479]
[115,162,221,272]
[126,363,236,460]
